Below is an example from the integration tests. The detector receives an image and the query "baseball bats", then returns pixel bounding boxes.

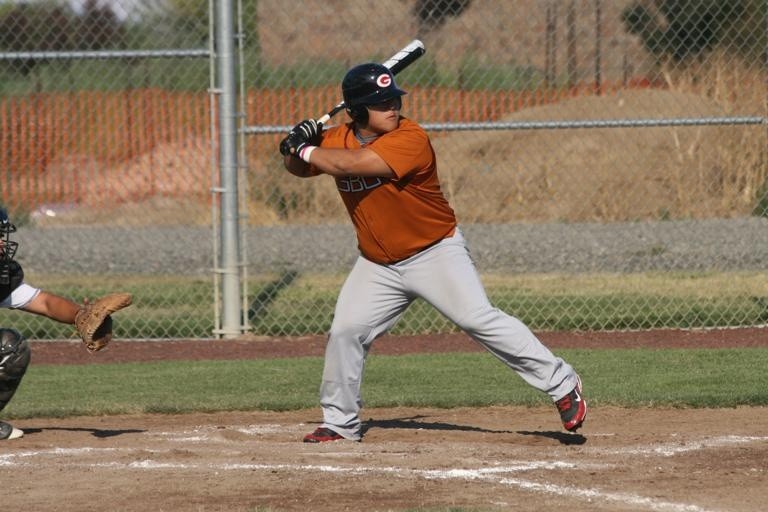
[316,39,425,126]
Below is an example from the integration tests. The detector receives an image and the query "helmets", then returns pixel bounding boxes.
[342,64,406,126]
[1,210,18,264]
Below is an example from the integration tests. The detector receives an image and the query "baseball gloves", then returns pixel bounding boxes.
[75,292,133,353]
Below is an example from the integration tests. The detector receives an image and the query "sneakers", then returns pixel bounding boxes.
[555,373,587,431]
[0,424,26,440]
[303,427,344,443]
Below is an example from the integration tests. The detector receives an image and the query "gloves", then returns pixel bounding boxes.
[292,118,322,142]
[282,134,309,156]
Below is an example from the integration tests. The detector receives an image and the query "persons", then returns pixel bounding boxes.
[0,206,134,444]
[285,62,587,443]
